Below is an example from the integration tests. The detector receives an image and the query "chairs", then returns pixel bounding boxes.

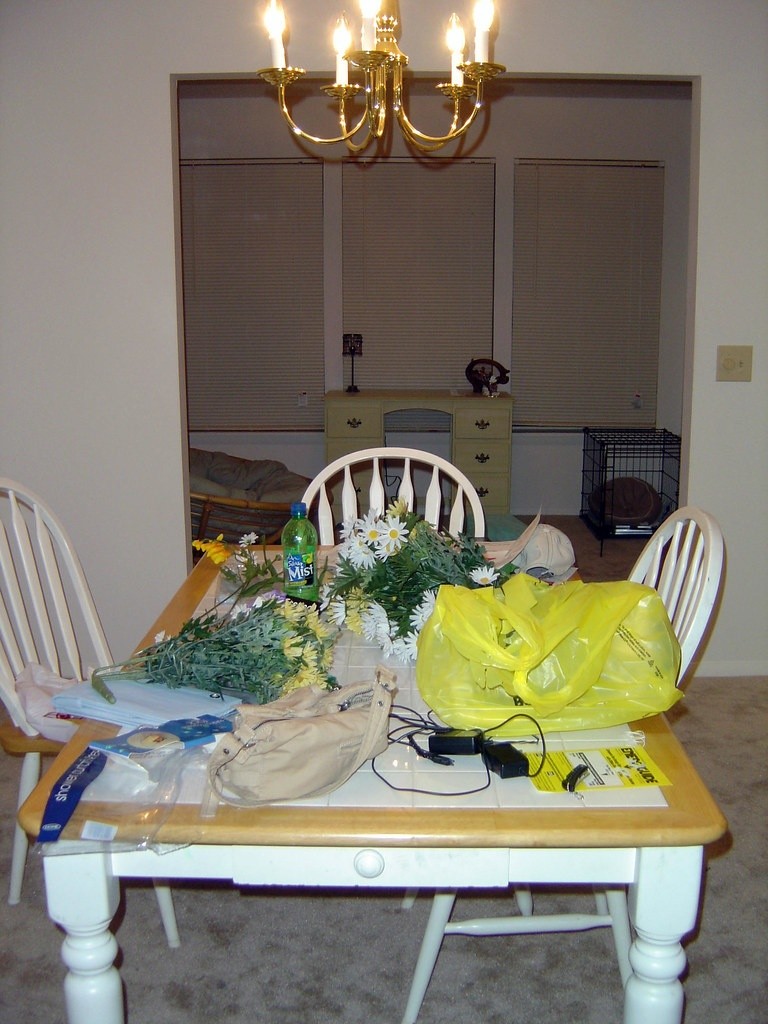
[402,506,725,1023]
[0,478,184,949]
[293,444,485,544]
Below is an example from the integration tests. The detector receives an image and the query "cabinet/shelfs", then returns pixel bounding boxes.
[324,390,512,524]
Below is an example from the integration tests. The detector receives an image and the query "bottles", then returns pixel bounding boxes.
[282,503,318,603]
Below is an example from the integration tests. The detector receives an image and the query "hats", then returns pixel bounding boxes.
[515,523,575,579]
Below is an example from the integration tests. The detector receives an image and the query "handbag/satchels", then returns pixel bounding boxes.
[210,664,398,805]
[417,572,684,738]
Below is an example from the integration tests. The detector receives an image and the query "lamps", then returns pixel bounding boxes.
[257,0,506,153]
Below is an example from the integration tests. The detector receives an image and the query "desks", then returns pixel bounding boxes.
[19,545,724,1024]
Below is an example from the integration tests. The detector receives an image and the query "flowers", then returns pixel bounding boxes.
[95,531,338,707]
[318,499,501,658]
